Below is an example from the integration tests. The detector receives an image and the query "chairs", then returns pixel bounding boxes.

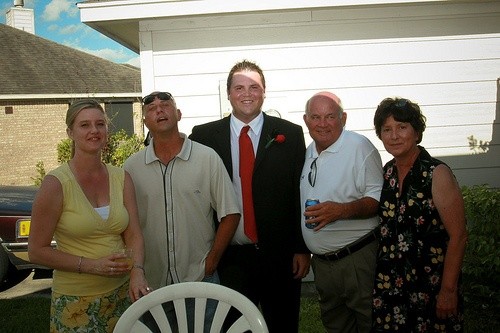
[113,281,269,333]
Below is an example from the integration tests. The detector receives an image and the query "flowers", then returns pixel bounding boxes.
[264,133,285,149]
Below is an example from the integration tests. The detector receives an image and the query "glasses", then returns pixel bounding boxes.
[308,157,317,187]
[141,92,171,104]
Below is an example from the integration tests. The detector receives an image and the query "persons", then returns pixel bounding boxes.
[187,61,310,333]
[299,91,384,333]
[371,98,470,333]
[27,98,153,333]
[119,90,242,333]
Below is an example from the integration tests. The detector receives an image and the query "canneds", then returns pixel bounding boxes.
[304,198,320,229]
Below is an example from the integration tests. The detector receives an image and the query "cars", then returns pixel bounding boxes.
[0,185,57,292]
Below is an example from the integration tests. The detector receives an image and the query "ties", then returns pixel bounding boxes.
[239,125,257,243]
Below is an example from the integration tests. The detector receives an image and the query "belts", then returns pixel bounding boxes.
[313,226,380,261]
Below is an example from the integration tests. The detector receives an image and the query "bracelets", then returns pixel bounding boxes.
[129,265,146,275]
[78,256,84,273]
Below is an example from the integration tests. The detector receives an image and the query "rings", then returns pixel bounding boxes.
[111,268,114,272]
